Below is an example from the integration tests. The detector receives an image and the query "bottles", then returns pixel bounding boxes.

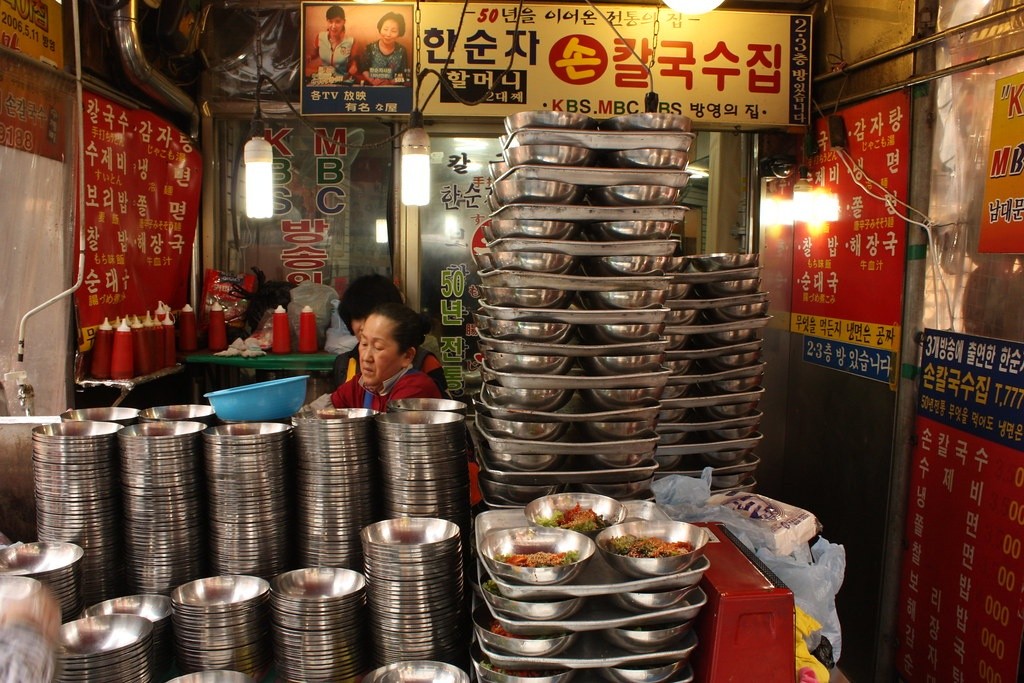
[297,306,318,354]
[208,301,228,352]
[91,300,178,379]
[272,306,290,354]
[180,304,198,352]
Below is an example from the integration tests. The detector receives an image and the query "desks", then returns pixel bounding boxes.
[186,350,337,405]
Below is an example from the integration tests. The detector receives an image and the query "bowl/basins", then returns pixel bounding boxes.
[3,373,710,683]
[476,102,761,520]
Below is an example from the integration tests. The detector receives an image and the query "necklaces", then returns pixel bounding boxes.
[329,37,338,43]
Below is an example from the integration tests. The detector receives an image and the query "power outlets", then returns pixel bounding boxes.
[830,115,846,148]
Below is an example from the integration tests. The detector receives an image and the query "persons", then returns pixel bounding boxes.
[358,12,407,86]
[305,5,360,84]
[330,275,452,398]
[297,304,482,505]
